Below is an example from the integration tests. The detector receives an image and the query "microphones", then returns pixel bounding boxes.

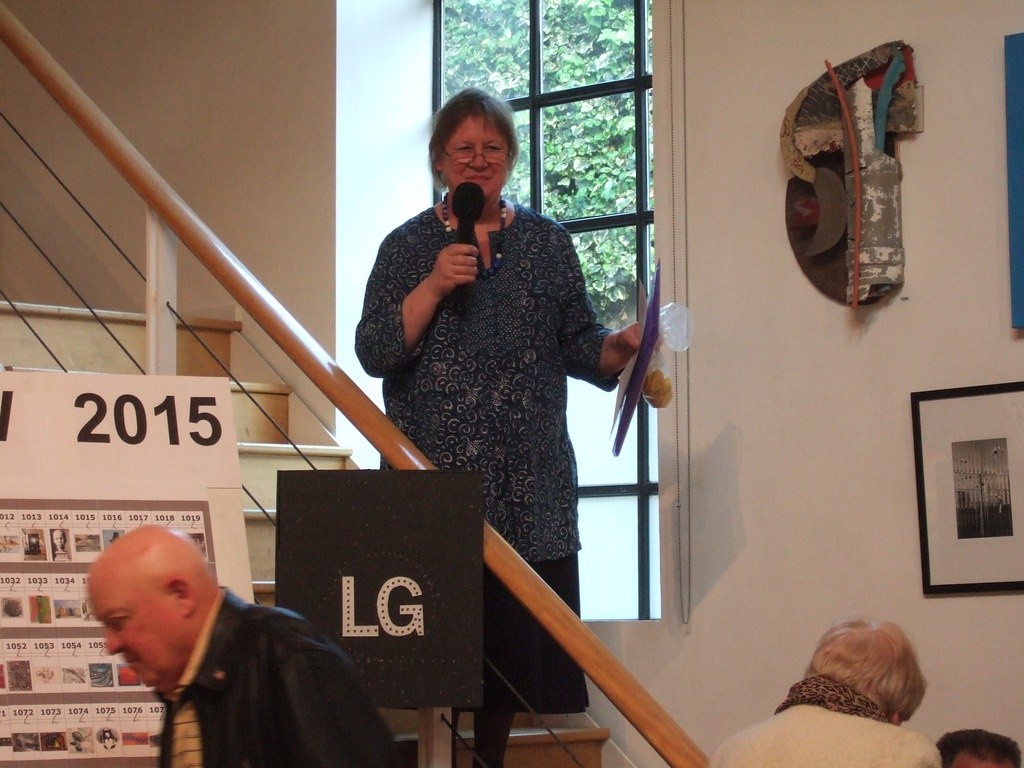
[451,182,483,303]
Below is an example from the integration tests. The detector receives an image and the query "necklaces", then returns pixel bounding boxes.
[442,193,507,279]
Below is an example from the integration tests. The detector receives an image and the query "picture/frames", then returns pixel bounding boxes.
[910,381,1024,597]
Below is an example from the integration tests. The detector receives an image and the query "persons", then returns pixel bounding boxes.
[711,618,1024,768]
[86,526,398,768]
[354,87,664,768]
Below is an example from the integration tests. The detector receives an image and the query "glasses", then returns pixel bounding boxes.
[441,144,511,166]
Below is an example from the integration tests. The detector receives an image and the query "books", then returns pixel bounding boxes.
[612,260,661,457]
